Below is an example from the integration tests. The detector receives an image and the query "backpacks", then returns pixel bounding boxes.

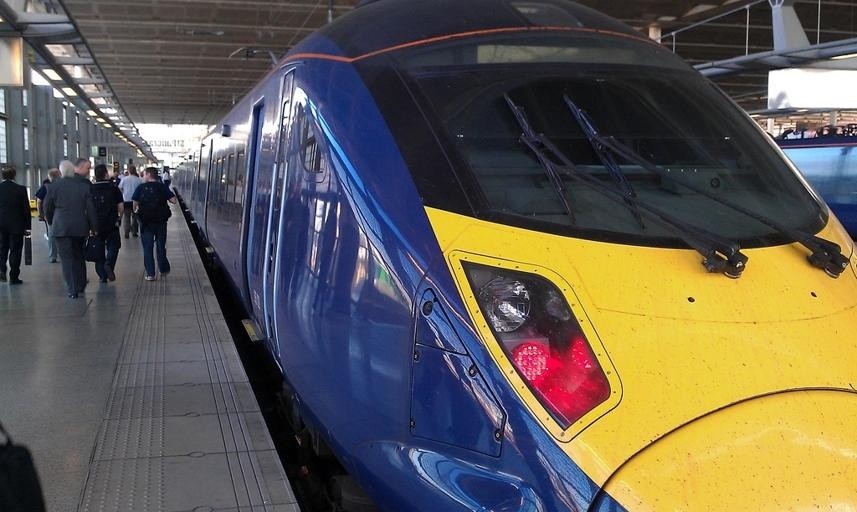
[139,183,164,225]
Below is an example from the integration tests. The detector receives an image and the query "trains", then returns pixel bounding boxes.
[173,0,857,512]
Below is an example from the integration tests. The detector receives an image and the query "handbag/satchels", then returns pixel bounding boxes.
[0,423,46,512]
[85,229,101,263]
[24,233,33,265]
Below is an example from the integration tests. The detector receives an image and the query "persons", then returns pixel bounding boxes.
[0,167,31,284]
[35,158,176,299]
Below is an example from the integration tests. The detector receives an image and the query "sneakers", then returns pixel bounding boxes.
[66,279,87,299]
[1,274,23,284]
[125,234,138,239]
[144,268,171,281]
[98,264,116,282]
